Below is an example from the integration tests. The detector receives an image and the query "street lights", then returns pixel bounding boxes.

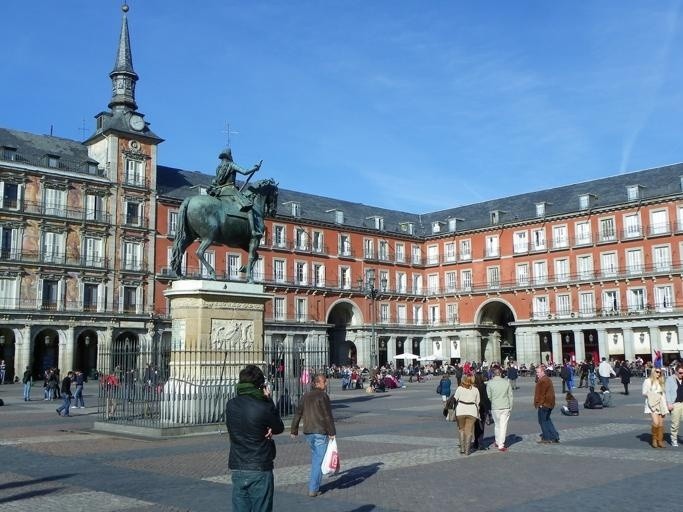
[356,267,387,370]
[149,313,164,385]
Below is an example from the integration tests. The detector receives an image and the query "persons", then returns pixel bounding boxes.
[0,359,6,385]
[22,365,32,401]
[289,373,336,497]
[325,364,366,390]
[561,392,579,416]
[225,364,284,512]
[473,372,490,451]
[485,368,513,452]
[532,366,560,445]
[452,373,480,456]
[561,356,652,394]
[584,385,616,409]
[36,363,164,422]
[640,366,669,449]
[206,147,264,237]
[463,356,557,390]
[370,361,463,397]
[664,364,683,448]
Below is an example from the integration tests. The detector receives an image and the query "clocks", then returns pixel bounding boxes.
[129,114,146,133]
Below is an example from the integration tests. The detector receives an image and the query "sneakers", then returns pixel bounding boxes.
[70,406,79,409]
[79,406,85,409]
[494,443,508,451]
[62,414,72,418]
[670,439,679,447]
[308,489,322,497]
[55,409,61,416]
[537,439,560,445]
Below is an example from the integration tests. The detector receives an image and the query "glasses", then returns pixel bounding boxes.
[655,371,660,373]
[678,372,683,374]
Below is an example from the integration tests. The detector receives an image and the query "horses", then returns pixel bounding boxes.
[169,177,279,285]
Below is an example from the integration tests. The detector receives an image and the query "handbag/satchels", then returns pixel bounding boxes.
[485,409,495,425]
[445,401,457,423]
[436,385,442,393]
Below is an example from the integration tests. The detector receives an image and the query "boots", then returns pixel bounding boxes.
[650,424,659,448]
[465,433,472,456]
[657,425,666,448]
[458,431,466,453]
